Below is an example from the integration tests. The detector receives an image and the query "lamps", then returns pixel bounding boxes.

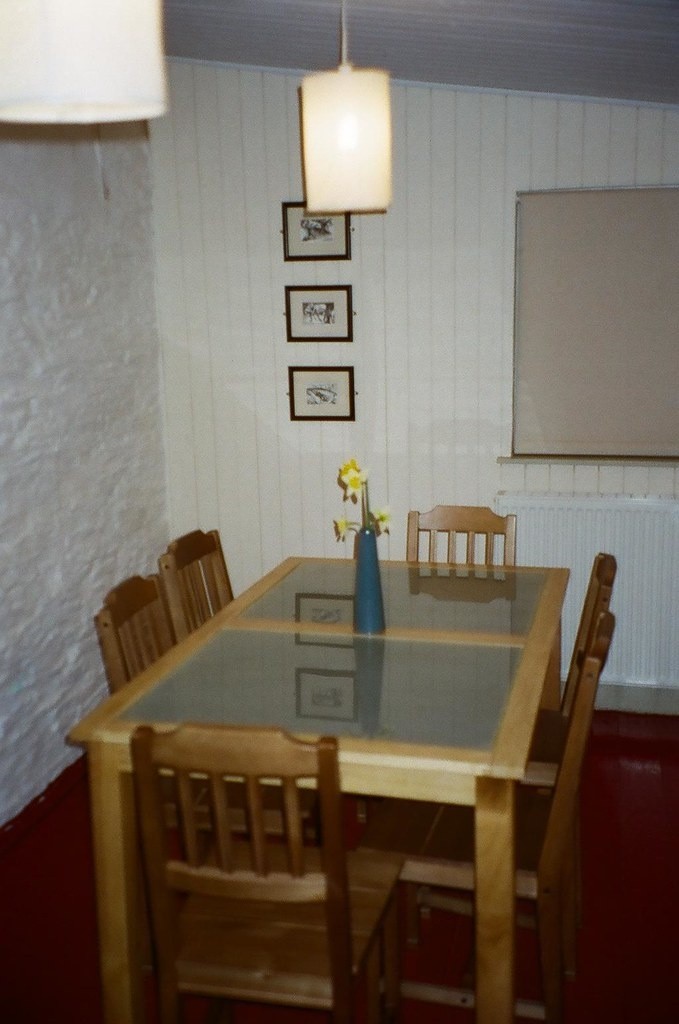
[0,0,169,125]
[301,0,393,214]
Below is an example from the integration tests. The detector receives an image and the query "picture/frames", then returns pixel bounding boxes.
[284,286,353,342]
[294,592,354,648]
[288,366,356,422]
[295,667,360,722]
[281,201,351,261]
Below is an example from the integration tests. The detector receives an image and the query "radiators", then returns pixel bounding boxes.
[496,490,679,690]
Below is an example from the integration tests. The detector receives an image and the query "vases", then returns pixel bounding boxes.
[355,528,384,633]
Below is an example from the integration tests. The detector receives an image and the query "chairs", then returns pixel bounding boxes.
[407,506,517,568]
[96,529,366,850]
[132,721,411,1024]
[520,552,616,974]
[360,611,616,1024]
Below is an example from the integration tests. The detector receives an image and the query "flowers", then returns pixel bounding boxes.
[334,459,389,537]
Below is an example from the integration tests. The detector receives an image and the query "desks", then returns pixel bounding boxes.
[65,556,569,1024]
